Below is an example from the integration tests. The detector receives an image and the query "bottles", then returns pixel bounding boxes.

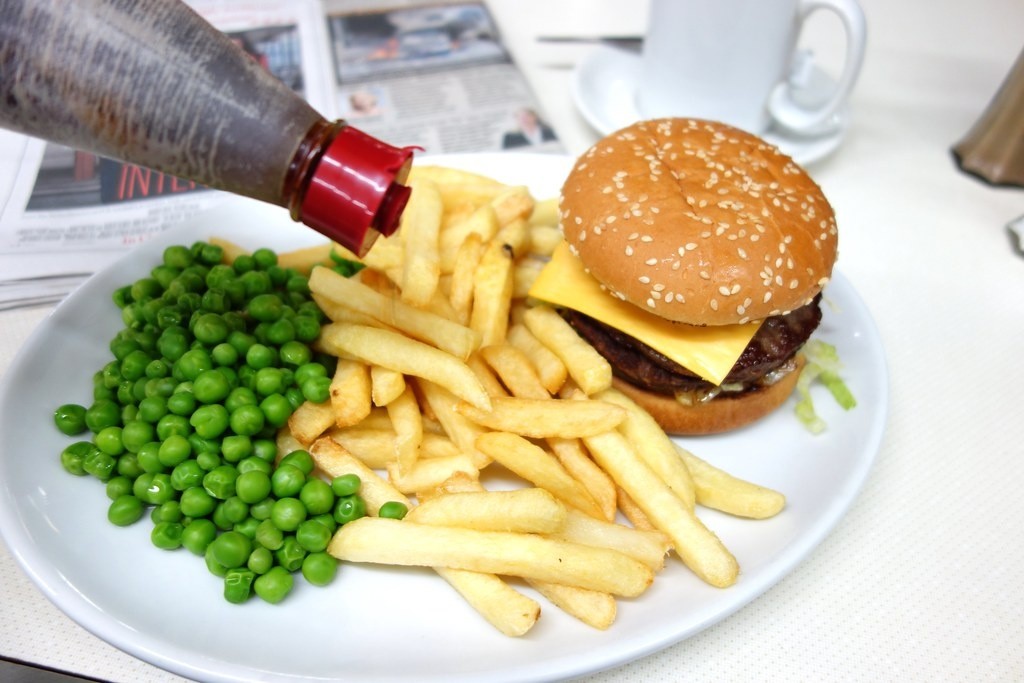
[0,0,414,261]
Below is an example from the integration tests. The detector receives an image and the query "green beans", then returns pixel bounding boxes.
[54,241,407,605]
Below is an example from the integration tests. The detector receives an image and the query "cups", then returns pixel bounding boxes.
[638,0,868,138]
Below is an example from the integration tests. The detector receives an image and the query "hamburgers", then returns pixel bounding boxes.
[528,118,840,436]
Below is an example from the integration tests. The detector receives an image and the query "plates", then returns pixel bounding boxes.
[0,148,891,683]
[571,37,845,166]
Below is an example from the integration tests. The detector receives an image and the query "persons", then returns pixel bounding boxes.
[345,91,381,120]
[228,32,270,72]
[502,107,556,151]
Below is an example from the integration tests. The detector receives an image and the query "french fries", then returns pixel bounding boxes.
[204,164,786,637]
